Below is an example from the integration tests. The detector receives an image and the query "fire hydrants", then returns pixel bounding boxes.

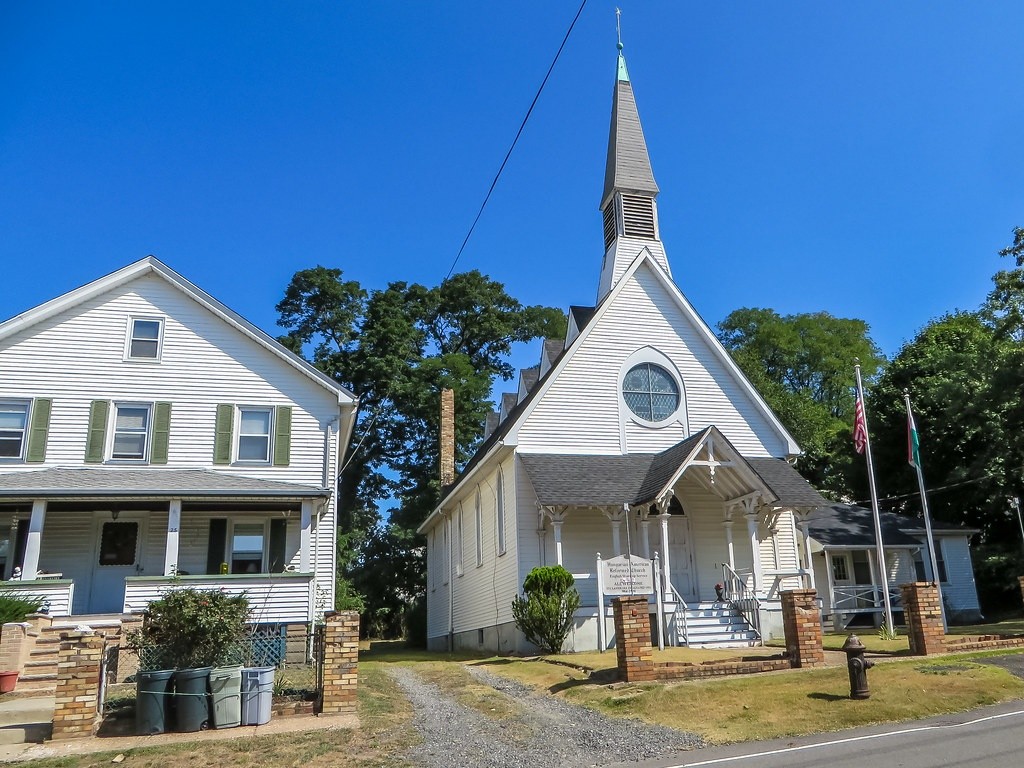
[841,633,875,700]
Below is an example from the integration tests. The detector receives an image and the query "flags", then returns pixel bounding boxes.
[851,377,867,455]
[905,399,922,470]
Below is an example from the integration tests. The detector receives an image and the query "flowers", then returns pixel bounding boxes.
[714,583,723,590]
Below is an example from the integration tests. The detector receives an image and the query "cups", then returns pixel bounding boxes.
[217,563,228,574]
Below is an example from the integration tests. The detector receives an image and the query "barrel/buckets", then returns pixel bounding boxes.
[0,672,18,693]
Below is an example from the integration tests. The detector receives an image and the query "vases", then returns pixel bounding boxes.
[714,589,725,602]
[0,671,18,693]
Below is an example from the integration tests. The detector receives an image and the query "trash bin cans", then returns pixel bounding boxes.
[132,668,174,735]
[174,666,213,732]
[241,666,276,727]
[209,663,244,729]
